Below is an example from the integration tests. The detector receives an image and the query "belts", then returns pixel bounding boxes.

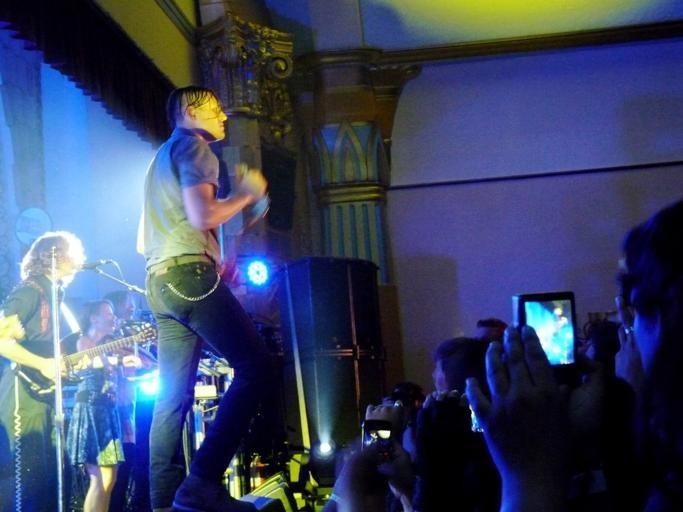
[74,388,117,406]
[144,254,216,277]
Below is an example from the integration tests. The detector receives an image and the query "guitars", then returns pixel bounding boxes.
[12,329,157,400]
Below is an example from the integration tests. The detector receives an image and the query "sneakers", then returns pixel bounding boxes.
[173,475,256,512]
[151,496,174,512]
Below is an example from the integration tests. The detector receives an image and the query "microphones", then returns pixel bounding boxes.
[240,162,270,218]
[79,259,112,269]
[135,309,152,317]
[116,318,145,328]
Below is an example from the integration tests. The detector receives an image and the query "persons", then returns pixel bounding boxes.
[63,296,135,511]
[135,87,277,511]
[0,229,94,511]
[318,199,683,510]
[103,290,152,510]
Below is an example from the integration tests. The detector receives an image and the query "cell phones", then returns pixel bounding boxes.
[512,290,578,386]
[361,419,394,464]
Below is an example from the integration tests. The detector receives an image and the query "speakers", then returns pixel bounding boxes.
[239,471,298,512]
